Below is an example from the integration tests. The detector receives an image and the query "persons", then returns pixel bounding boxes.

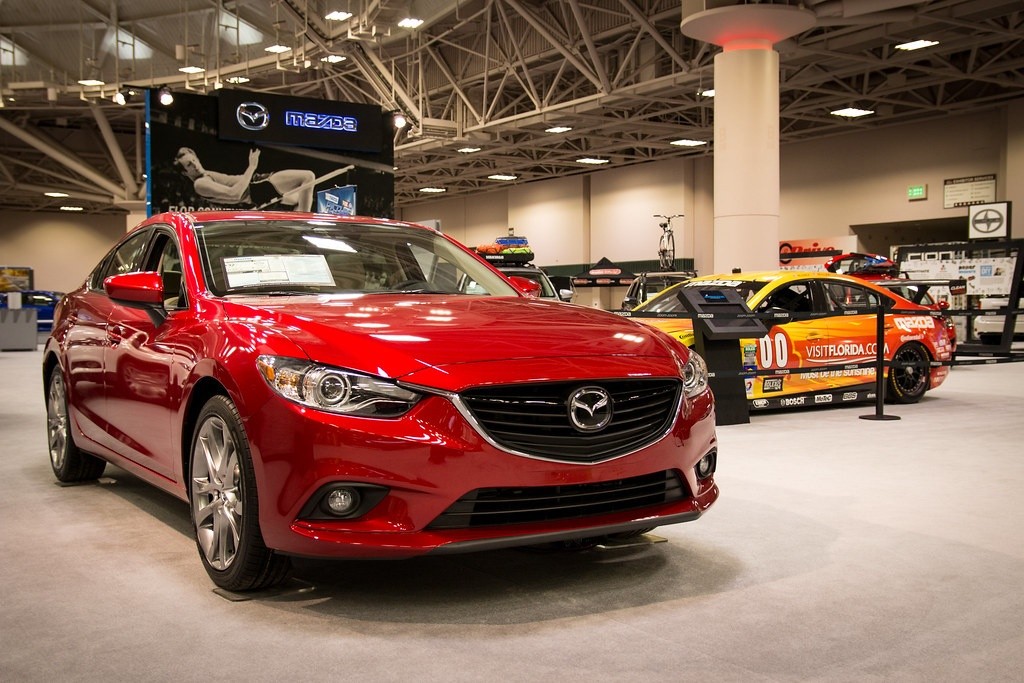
[173,148,315,212]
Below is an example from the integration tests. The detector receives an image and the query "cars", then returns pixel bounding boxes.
[0,290,66,332]
[494,264,574,303]
[42,211,720,592]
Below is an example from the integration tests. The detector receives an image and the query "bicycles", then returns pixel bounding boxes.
[653,215,685,271]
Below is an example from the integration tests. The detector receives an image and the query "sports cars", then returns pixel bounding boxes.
[622,272,957,412]
